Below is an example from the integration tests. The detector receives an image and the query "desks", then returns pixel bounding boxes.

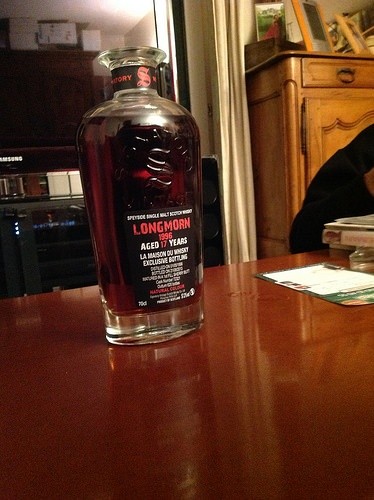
[0,199,87,299]
[0,248,374,500]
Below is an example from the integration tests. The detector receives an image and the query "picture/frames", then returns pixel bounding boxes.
[254,1,290,41]
[333,14,371,55]
[291,0,335,53]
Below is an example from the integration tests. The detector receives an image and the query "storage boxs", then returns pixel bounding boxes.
[9,33,38,49]
[37,22,78,46]
[9,17,39,33]
[81,29,103,52]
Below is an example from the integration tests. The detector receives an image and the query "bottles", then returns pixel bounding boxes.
[75,45,206,346]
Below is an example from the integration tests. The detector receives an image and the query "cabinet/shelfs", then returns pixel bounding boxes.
[245,49,374,260]
[0,49,104,148]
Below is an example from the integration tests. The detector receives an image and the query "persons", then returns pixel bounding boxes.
[324,231,341,244]
[289,123,374,254]
[263,11,283,40]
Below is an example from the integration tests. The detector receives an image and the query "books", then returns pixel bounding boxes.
[322,214,374,247]
